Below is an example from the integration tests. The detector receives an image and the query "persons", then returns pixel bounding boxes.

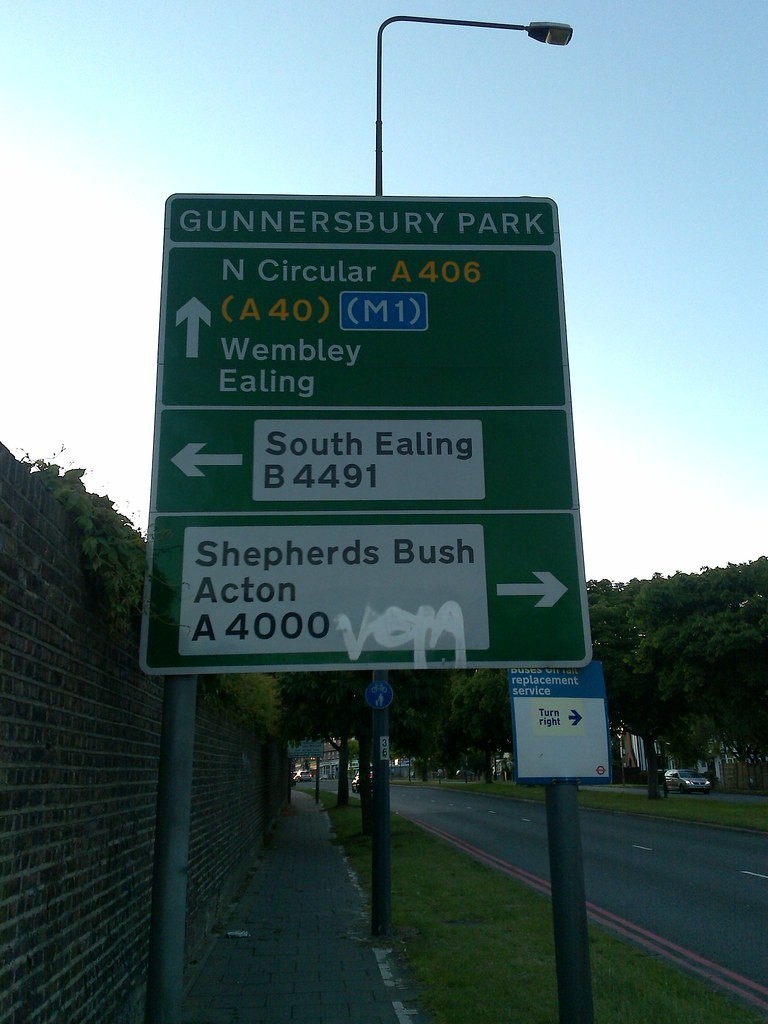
[749,776,755,790]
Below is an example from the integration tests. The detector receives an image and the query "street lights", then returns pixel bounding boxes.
[368,15,576,949]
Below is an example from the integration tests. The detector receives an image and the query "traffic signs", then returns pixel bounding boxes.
[508,659,614,786]
[135,197,594,676]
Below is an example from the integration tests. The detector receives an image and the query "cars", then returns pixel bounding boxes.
[456,770,474,777]
[293,771,312,783]
[351,771,373,793]
[664,769,712,795]
